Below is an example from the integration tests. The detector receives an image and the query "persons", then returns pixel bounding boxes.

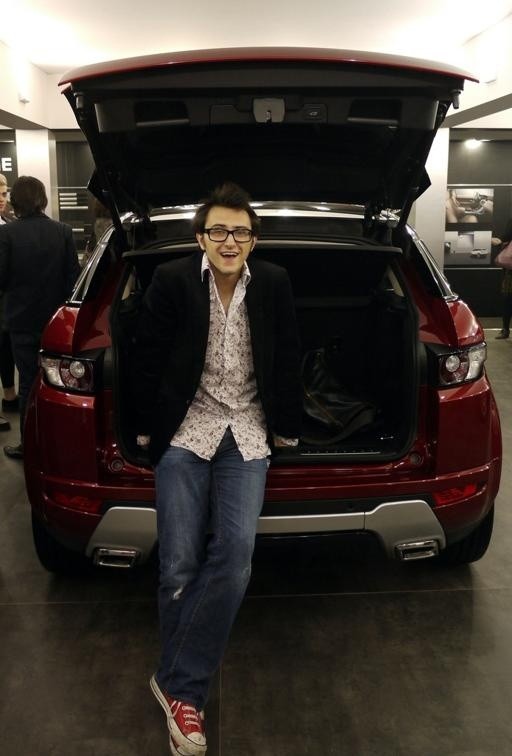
[0,171,24,432]
[0,174,81,460]
[80,192,115,269]
[111,177,307,754]
[489,214,512,340]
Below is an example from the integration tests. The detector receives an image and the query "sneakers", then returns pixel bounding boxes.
[495,330,509,338]
[149,670,208,756]
[4,446,25,459]
[0,395,23,431]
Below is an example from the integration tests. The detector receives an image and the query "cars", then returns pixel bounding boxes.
[470,247,488,258]
[24,47,502,579]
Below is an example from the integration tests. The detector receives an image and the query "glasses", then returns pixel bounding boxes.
[201,226,256,244]
